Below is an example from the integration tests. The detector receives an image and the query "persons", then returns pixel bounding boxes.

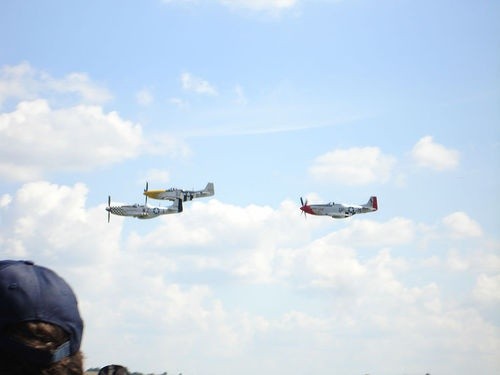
[99,364,131,374]
[0,259,84,375]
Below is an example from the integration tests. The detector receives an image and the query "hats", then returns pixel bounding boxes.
[0,258,84,368]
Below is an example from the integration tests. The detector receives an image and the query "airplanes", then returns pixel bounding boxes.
[300,194,378,218]
[104,195,183,224]
[142,181,214,205]
